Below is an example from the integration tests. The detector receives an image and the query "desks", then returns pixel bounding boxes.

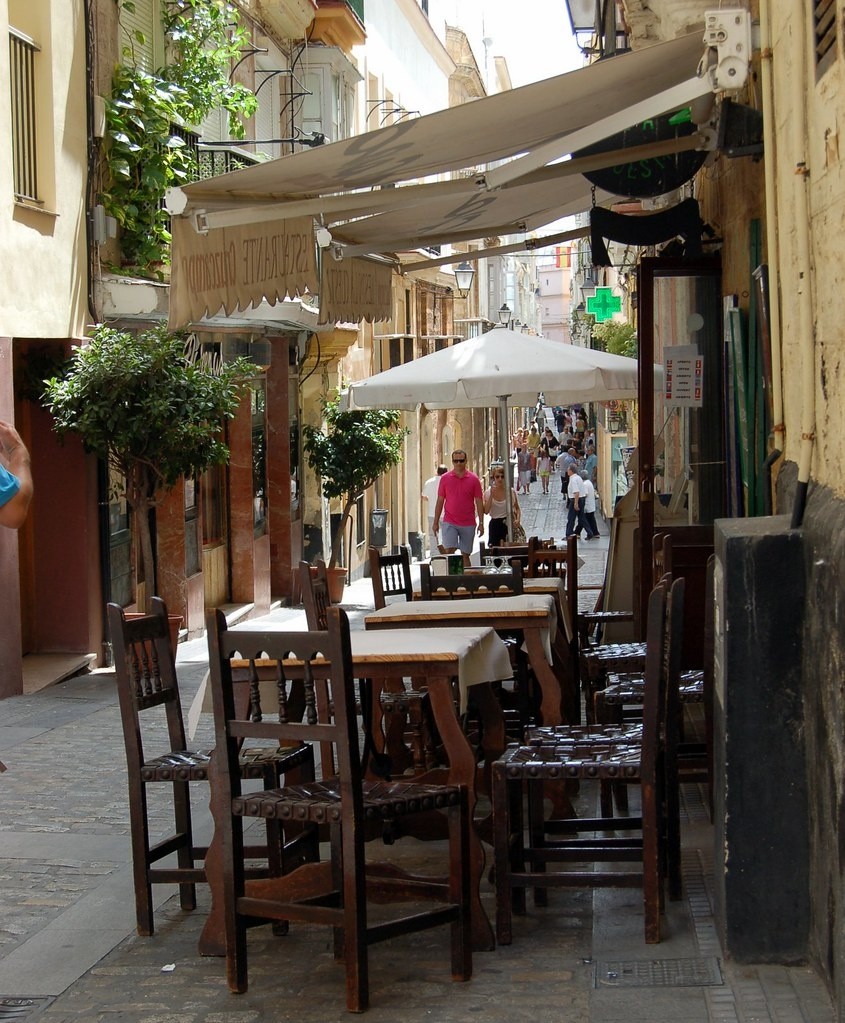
[189,627,514,951]
[365,593,580,835]
[523,539,586,572]
[414,577,574,645]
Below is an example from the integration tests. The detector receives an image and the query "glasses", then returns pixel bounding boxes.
[453,459,466,463]
[495,475,504,479]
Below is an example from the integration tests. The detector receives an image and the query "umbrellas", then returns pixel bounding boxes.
[336,327,665,543]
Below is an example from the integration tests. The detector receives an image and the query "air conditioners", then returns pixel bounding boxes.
[251,337,272,372]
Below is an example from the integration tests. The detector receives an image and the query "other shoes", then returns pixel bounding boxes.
[523,491,530,495]
[562,537,567,541]
[543,491,548,495]
[585,536,591,540]
[576,534,581,539]
[593,534,600,538]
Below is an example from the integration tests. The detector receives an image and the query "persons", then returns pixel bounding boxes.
[512,403,627,540]
[482,467,521,568]
[432,449,483,567]
[420,464,448,565]
[0,421,33,529]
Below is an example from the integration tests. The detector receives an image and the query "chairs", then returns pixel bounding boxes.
[108,532,716,1013]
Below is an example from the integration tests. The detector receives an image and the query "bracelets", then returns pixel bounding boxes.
[479,521,482,525]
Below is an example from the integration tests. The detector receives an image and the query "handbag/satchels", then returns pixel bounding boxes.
[594,489,599,499]
[513,524,526,543]
[517,473,521,491]
[549,448,557,457]
[530,471,534,482]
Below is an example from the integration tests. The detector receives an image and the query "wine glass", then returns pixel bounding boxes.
[499,556,512,575]
[483,556,500,575]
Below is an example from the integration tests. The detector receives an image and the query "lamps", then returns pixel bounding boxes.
[434,261,475,309]
[199,132,331,148]
[717,97,765,162]
[571,302,586,340]
[609,417,629,434]
[484,304,529,335]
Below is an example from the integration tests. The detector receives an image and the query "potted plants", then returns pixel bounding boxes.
[299,376,412,604]
[40,318,263,674]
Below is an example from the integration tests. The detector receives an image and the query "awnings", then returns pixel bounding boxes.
[163,29,709,325]
